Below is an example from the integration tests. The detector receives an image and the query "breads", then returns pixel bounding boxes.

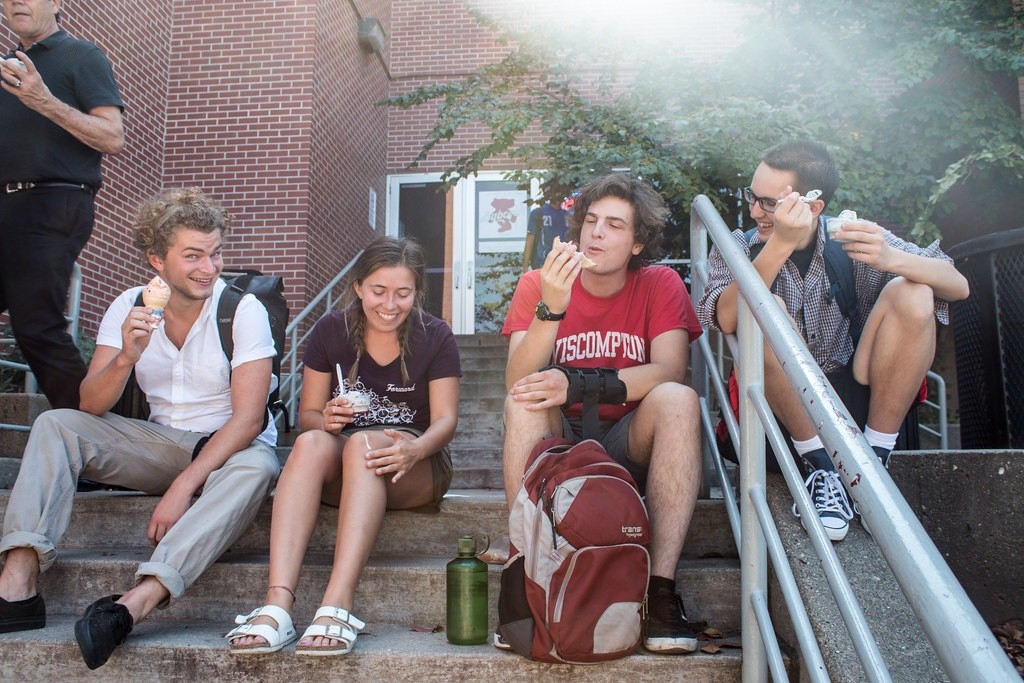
[553,235,596,269]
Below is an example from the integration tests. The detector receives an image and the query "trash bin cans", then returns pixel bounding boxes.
[946,228,1024,449]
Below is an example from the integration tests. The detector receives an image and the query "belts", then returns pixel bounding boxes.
[5,181,93,194]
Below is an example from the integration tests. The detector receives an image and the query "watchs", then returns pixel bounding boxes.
[534,301,567,321]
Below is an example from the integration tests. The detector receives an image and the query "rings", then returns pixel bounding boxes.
[16,81,21,87]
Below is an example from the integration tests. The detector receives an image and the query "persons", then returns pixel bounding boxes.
[493,174,703,654]
[695,139,969,542]
[0,0,125,491]
[0,187,279,671]
[224,235,462,656]
[522,198,573,273]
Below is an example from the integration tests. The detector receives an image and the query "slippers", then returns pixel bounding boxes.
[295,606,366,656]
[224,605,297,654]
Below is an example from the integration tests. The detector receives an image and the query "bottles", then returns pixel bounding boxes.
[446,532,490,645]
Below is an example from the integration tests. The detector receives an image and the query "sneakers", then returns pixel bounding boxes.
[0,592,46,633]
[791,447,854,541]
[494,623,512,651]
[641,588,699,655]
[74,594,133,670]
[854,445,891,535]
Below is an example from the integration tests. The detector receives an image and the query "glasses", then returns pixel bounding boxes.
[744,186,777,213]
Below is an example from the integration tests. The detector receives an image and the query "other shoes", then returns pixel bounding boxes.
[76,477,140,492]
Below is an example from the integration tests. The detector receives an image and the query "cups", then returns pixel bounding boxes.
[826,219,852,242]
[1,61,26,75]
[341,402,369,412]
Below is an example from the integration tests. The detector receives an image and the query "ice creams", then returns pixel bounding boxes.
[338,391,369,412]
[142,275,171,310]
[0,57,28,76]
[825,209,860,244]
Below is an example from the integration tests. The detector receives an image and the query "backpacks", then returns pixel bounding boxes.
[132,270,290,413]
[499,367,652,665]
[715,215,927,467]
